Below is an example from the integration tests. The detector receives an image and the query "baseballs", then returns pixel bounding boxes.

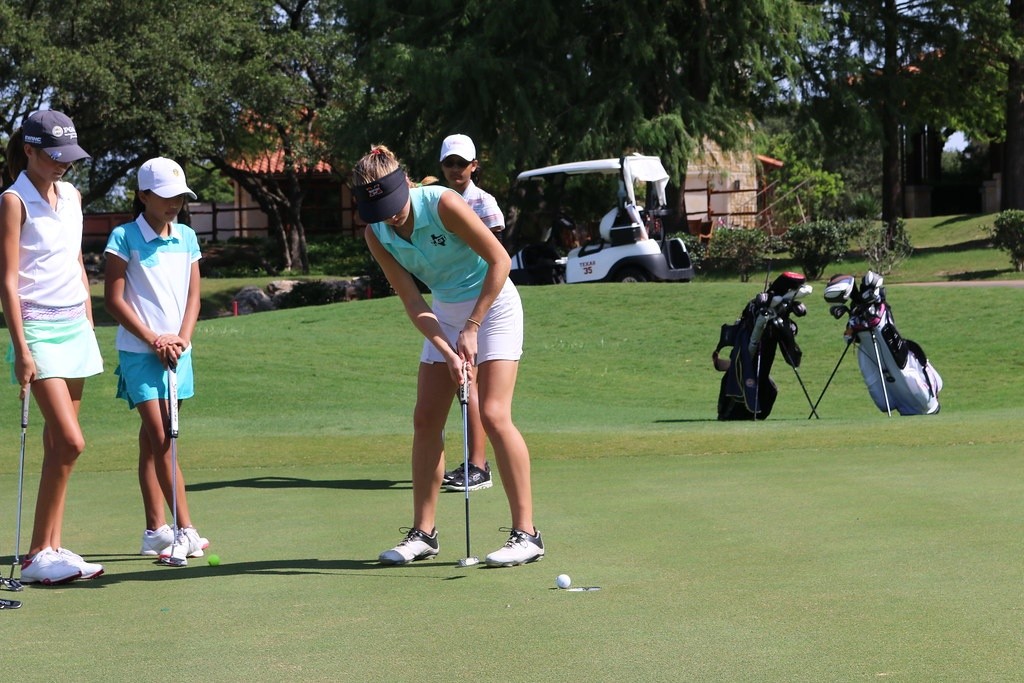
[558,574,571,589]
[208,555,220,566]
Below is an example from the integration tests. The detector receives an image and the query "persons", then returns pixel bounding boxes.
[440,134,505,490]
[104,157,209,560]
[0,109,104,584]
[353,144,545,566]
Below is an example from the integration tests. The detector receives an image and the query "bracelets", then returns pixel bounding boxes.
[467,318,481,327]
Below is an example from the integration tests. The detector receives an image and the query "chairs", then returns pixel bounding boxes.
[687,219,714,255]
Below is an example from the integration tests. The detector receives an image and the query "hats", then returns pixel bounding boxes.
[138,156,198,200]
[440,134,475,161]
[22,109,91,163]
[352,168,409,223]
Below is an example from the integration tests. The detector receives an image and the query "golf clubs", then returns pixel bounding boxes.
[2,381,32,592]
[829,305,850,319]
[460,361,479,566]
[773,299,807,328]
[161,357,185,566]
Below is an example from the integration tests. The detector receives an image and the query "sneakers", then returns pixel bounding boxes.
[378,527,440,565]
[20,547,104,583]
[158,527,204,566]
[139,524,209,556]
[486,527,545,566]
[441,459,493,492]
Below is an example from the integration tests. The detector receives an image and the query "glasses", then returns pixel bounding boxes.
[442,158,472,169]
